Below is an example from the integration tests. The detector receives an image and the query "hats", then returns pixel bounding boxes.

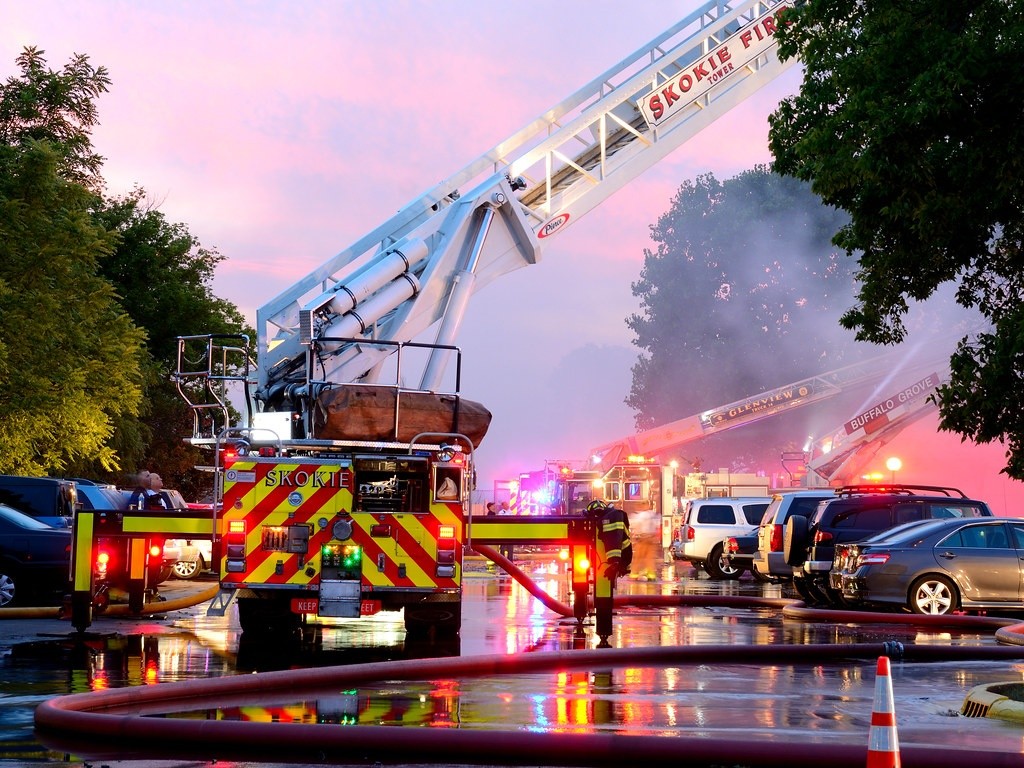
[487,503,495,508]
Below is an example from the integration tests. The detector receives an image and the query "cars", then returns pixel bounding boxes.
[0,502,78,608]
[830,516,1024,619]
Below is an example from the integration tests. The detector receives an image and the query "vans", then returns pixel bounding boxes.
[0,475,80,520]
[120,486,189,511]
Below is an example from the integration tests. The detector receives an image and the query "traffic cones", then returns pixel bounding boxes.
[865,656,902,768]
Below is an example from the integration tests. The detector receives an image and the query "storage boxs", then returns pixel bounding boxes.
[252,411,292,440]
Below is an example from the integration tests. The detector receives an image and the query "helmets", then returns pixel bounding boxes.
[587,500,606,511]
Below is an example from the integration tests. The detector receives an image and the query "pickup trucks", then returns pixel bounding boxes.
[669,496,771,580]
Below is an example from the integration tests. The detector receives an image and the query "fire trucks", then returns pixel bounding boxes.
[168,0,830,633]
[514,329,966,548]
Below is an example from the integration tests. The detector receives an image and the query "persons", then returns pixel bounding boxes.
[487,502,517,572]
[131,469,167,601]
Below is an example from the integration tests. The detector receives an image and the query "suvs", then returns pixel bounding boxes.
[163,536,215,579]
[65,478,128,514]
[752,488,840,586]
[782,483,998,609]
